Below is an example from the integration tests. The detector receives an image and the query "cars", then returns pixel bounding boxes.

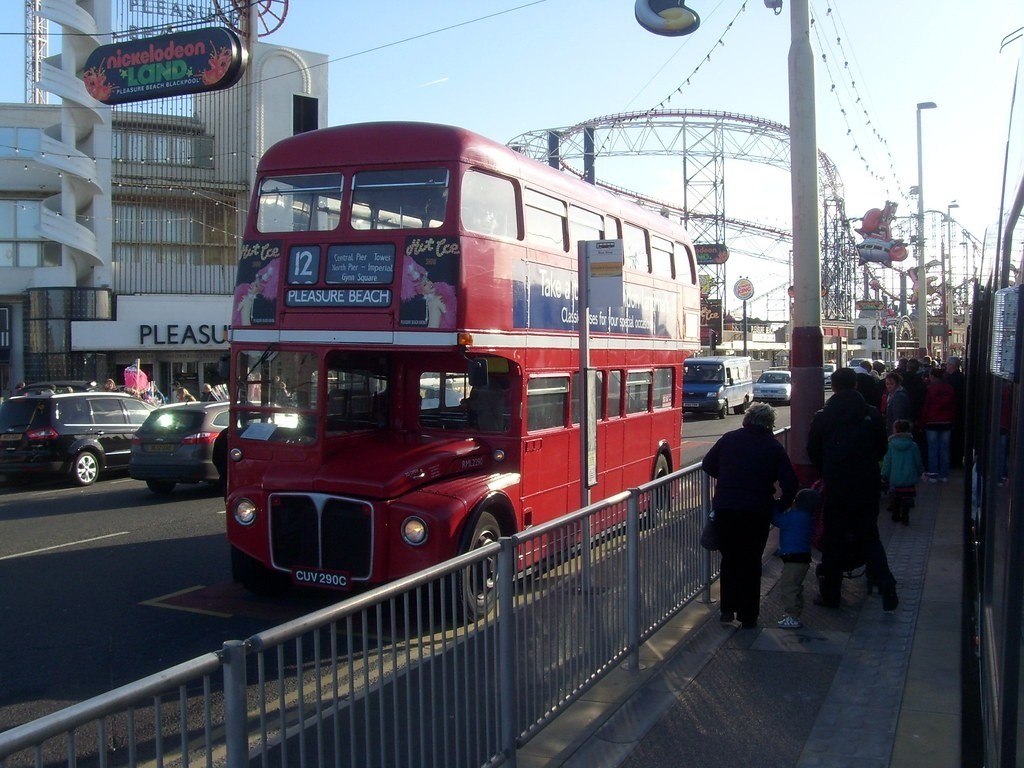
[752,369,791,405]
[824,363,836,384]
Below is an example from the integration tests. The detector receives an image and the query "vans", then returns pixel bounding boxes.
[680,354,754,419]
[848,358,873,371]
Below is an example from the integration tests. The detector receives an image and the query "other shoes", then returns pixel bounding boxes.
[812,594,841,608]
[942,477,948,483]
[777,619,802,627]
[928,478,937,484]
[891,514,900,522]
[719,613,736,625]
[879,583,899,611]
[901,516,909,525]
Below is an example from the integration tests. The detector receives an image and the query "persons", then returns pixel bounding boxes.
[415,278,447,328]
[16,369,289,504]
[237,280,263,326]
[702,356,966,631]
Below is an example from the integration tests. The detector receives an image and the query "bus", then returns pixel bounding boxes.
[225,118,700,626]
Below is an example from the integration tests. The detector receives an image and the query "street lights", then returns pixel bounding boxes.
[960,241,969,349]
[916,101,938,363]
[947,204,960,357]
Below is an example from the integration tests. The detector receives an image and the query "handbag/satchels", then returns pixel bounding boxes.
[700,510,719,551]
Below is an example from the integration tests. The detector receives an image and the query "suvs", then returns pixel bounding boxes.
[15,379,103,396]
[0,388,185,487]
[129,398,299,492]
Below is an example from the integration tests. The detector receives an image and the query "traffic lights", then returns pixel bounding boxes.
[880,329,888,348]
[888,331,895,349]
